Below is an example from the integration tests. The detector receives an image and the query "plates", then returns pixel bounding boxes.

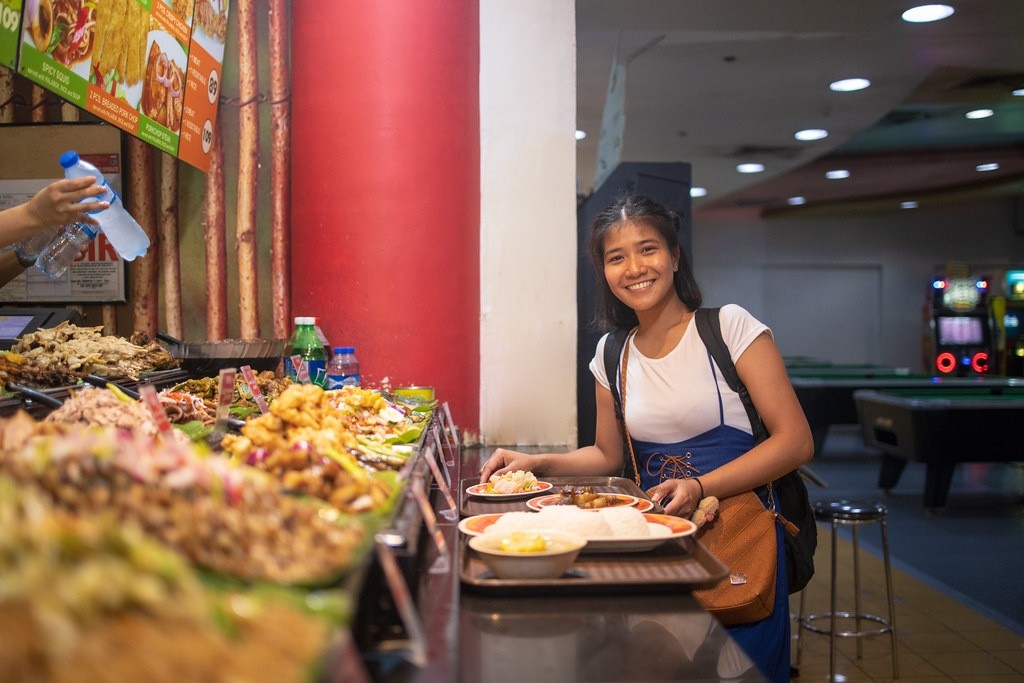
[466,481,554,500]
[121,30,188,136]
[526,493,655,513]
[459,513,697,554]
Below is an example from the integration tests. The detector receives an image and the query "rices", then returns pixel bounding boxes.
[489,504,655,546]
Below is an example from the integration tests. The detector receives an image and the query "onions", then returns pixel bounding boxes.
[153,53,182,96]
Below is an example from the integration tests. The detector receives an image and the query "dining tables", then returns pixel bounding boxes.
[459,445,766,683]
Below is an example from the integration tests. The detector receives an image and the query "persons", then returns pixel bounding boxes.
[0,176,111,288]
[576,612,753,683]
[479,194,814,683]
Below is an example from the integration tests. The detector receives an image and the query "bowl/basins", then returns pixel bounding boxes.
[468,534,588,579]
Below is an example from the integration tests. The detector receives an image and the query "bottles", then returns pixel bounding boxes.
[328,348,345,389]
[344,348,359,387]
[35,212,101,279]
[313,317,332,363]
[59,150,151,261]
[291,317,328,391]
[284,317,303,379]
[12,223,66,261]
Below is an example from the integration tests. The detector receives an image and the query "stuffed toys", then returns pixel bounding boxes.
[691,496,720,532]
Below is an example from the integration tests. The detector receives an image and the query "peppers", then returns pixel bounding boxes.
[48,5,119,96]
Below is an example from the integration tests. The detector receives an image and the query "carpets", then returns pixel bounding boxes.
[786,422,1024,631]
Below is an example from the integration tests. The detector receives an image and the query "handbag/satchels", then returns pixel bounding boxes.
[686,491,800,626]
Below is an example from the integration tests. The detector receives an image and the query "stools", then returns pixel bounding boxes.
[797,498,897,683]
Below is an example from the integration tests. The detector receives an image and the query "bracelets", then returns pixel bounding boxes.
[14,251,30,267]
[692,478,704,497]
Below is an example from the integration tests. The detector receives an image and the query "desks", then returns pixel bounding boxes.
[786,361,1024,517]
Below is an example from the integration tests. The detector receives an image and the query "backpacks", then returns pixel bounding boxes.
[607,306,817,596]
